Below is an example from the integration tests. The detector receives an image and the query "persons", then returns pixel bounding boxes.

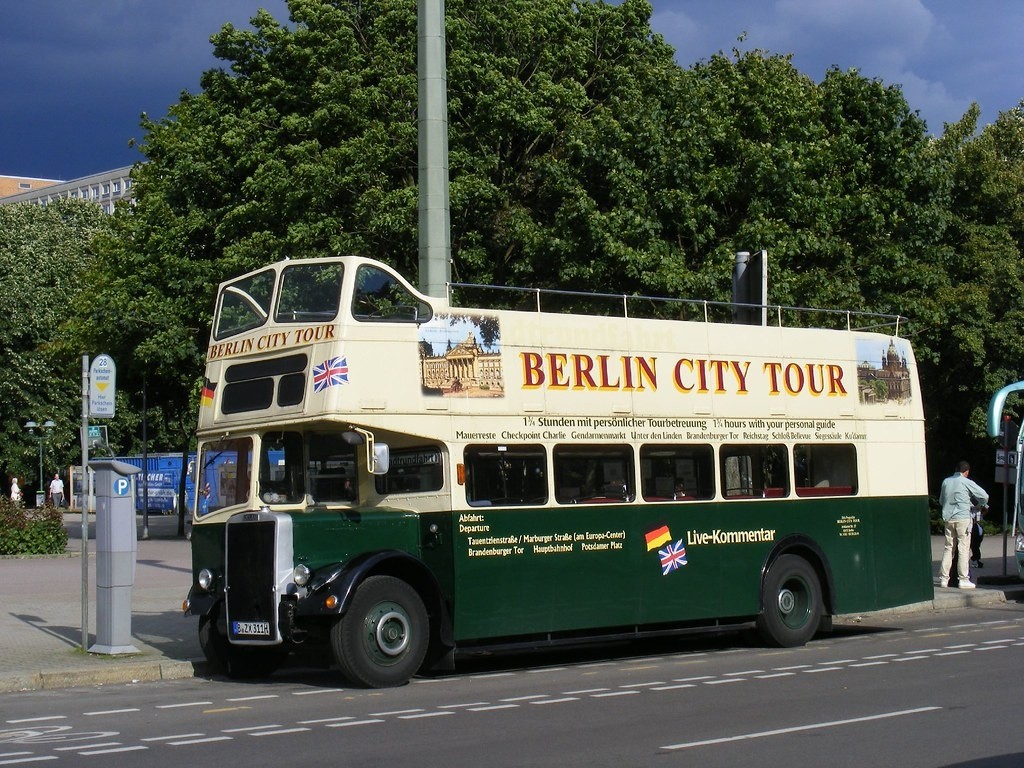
[10,477,21,501]
[938,460,992,590]
[49,473,65,510]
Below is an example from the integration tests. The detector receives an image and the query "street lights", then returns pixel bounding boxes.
[24,420,56,508]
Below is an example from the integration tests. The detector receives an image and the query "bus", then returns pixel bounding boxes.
[178,254,933,689]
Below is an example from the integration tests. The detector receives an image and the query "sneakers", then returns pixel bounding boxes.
[959,578,976,589]
[940,577,949,587]
